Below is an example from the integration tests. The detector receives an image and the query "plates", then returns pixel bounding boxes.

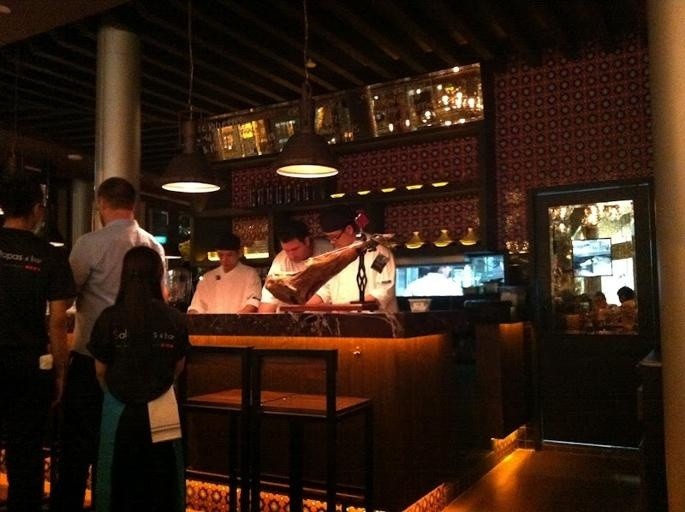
[244,240,268,254]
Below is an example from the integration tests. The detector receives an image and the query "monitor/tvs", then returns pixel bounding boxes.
[572,237,612,278]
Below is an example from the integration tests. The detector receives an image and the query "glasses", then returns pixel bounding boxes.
[325,228,347,242]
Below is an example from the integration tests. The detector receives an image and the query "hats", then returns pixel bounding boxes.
[319,203,355,234]
[216,233,243,251]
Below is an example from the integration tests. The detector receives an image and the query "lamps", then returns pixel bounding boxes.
[32,89,64,248]
[160,1,221,194]
[276,1,339,178]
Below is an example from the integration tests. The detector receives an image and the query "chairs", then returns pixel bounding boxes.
[251,347,373,511]
[181,346,296,511]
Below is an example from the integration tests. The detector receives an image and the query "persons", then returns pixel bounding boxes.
[552,287,638,332]
[47,178,167,511]
[307,205,396,314]
[186,233,262,315]
[257,220,334,312]
[402,263,464,297]
[87,246,193,512]
[0,173,77,511]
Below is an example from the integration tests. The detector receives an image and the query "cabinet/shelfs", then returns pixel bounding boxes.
[634,348,668,511]
[139,188,191,260]
[189,122,495,268]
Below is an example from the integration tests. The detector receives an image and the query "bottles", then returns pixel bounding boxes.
[250,177,322,208]
